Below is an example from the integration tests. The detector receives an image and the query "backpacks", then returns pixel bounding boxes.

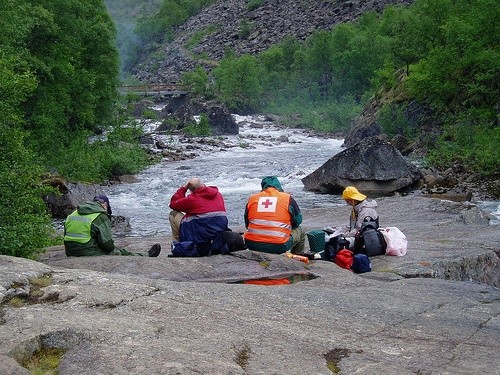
[208,231,244,256]
[360,224,387,256]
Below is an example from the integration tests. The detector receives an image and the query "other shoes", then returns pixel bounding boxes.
[149,243,160,257]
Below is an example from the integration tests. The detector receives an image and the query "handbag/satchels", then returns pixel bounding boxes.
[173,241,199,257]
[336,249,372,274]
[377,227,407,256]
[361,216,376,232]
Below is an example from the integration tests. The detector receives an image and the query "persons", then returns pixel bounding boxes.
[244,176,305,255]
[340,186,379,250]
[64,194,161,257]
[169,178,229,243]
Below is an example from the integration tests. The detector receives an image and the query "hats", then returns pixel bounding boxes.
[342,186,366,201]
[260,176,283,191]
[93,195,111,214]
